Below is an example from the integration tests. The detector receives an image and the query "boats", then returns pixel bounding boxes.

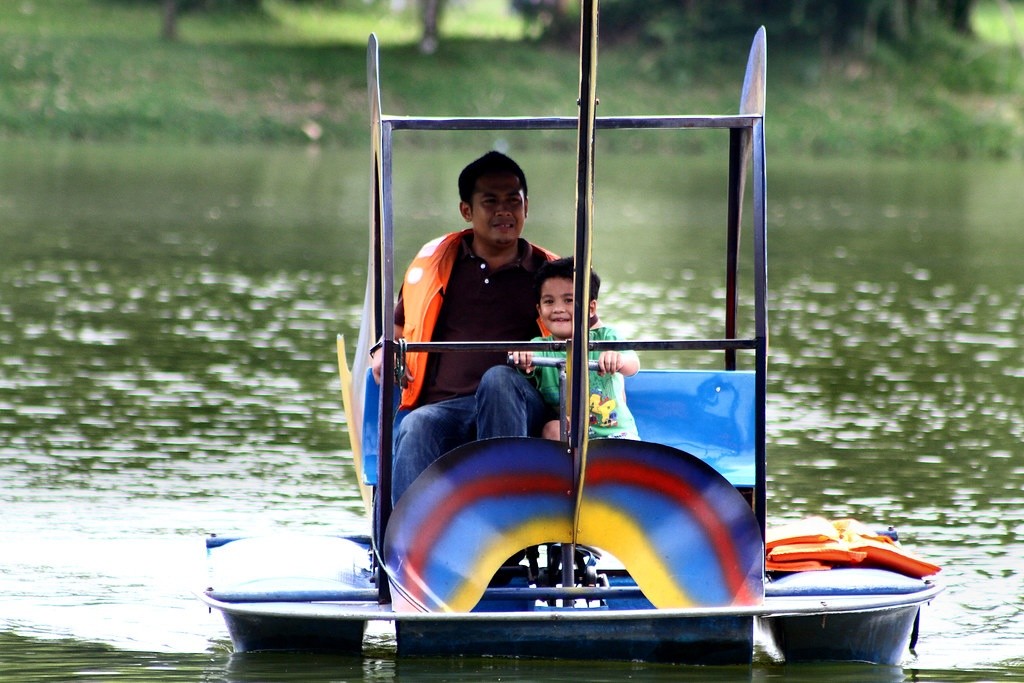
[193,24,945,668]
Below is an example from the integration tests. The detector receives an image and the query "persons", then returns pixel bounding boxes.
[370,150,560,507]
[507,255,639,444]
